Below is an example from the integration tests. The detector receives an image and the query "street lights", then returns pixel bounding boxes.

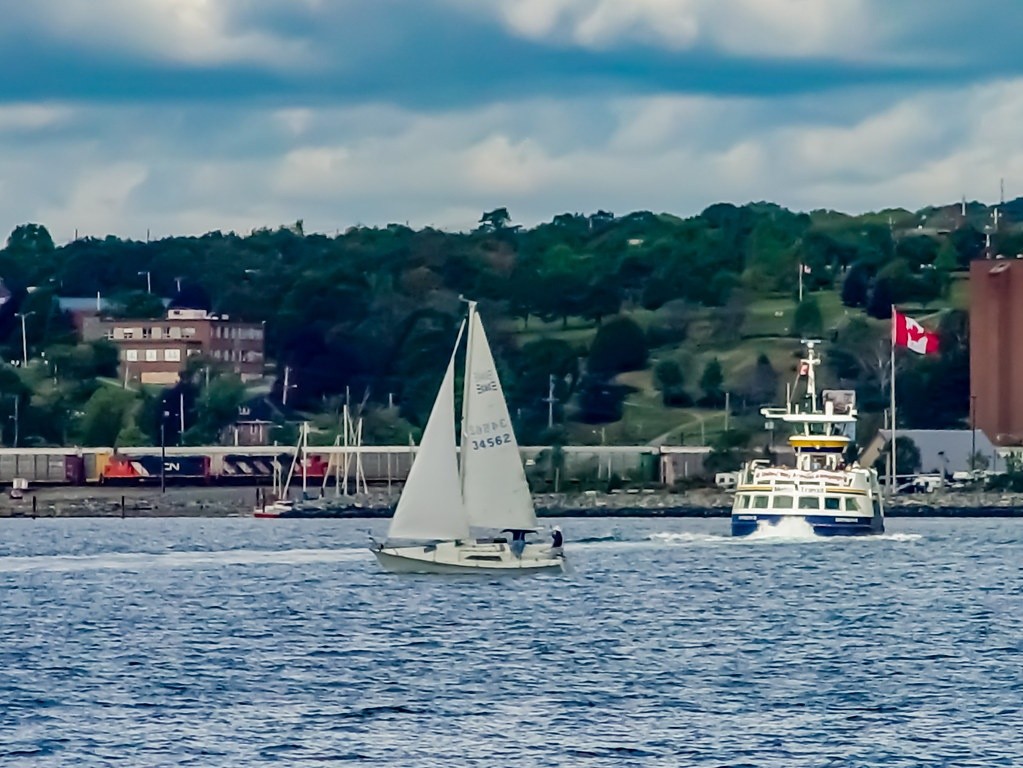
[137,272,151,294]
[14,311,36,368]
[9,394,19,448]
[969,395,978,471]
[282,366,298,404]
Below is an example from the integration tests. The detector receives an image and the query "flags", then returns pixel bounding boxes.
[892,310,939,356]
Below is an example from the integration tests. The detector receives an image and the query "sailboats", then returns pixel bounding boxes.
[731,338,886,537]
[254,384,396,517]
[368,295,565,574]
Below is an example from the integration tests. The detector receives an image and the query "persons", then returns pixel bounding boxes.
[551,525,562,549]
[813,458,846,472]
[500,529,539,560]
